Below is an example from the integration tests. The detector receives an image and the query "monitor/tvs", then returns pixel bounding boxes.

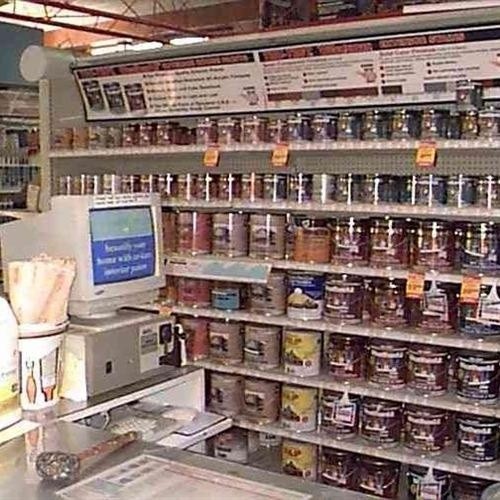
[0,191,166,331]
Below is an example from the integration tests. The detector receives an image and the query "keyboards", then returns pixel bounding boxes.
[103,415,184,443]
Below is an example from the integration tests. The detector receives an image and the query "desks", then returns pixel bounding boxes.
[1,364,234,451]
[1,421,399,500]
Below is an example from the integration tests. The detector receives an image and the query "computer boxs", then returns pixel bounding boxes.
[60,309,175,401]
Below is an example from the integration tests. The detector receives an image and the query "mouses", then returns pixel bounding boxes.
[162,407,198,421]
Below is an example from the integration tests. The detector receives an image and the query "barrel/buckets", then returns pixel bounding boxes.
[319,276,499,500]
[161,265,319,484]
[14,321,70,411]
[0,296,23,439]
[47,79,500,150]
[159,210,500,278]
[49,170,499,207]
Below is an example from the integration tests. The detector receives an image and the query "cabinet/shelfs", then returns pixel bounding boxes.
[40,6,500,500]
[0,21,43,225]
